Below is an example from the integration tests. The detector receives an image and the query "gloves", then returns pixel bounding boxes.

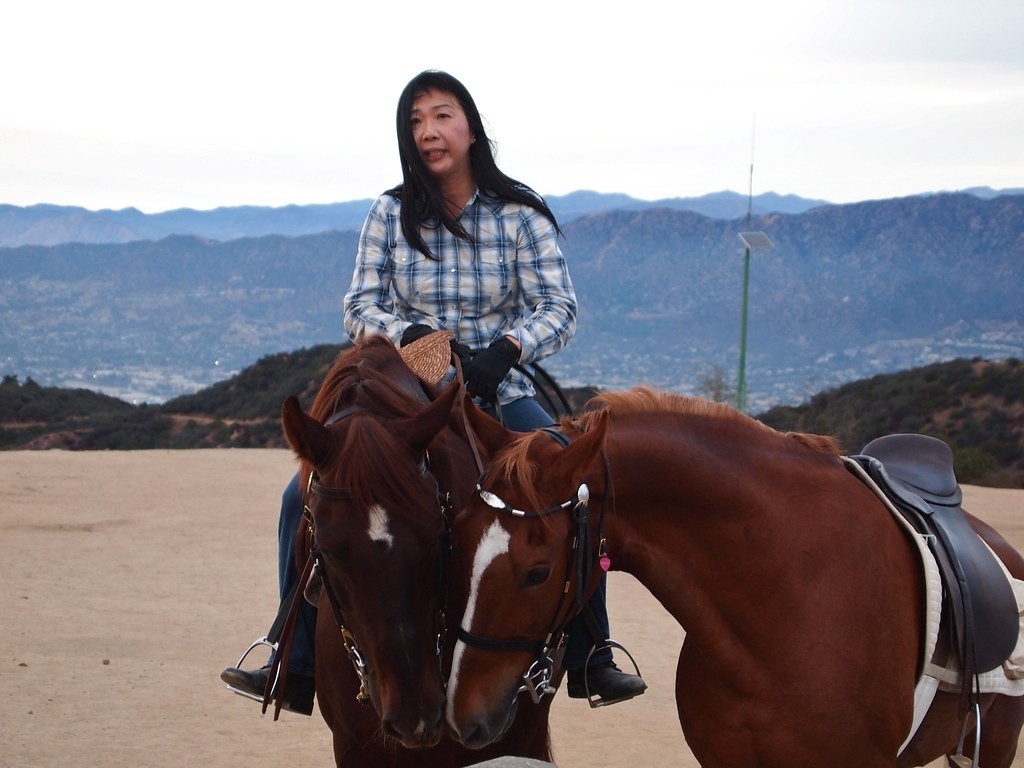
[400,321,471,370]
[463,335,522,408]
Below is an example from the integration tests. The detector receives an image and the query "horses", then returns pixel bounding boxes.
[441,384,1024,767]
[280,331,565,768]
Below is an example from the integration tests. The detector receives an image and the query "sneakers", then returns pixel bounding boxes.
[221,667,317,716]
[568,661,646,698]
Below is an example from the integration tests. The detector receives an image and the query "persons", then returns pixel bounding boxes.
[220,70,648,716]
